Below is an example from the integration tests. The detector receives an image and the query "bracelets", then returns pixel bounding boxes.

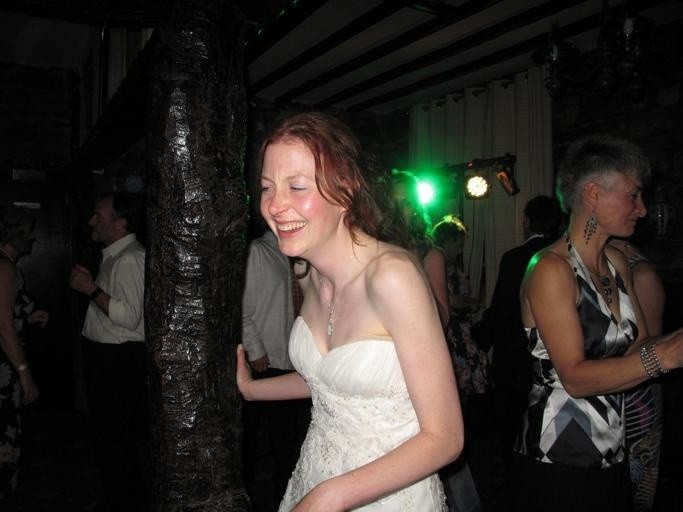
[640,343,672,378]
[88,287,103,300]
[16,362,28,371]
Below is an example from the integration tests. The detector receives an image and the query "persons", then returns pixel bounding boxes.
[520,133,682,512]
[380,169,570,512]
[237,113,465,512]
[240,230,304,512]
[606,237,666,512]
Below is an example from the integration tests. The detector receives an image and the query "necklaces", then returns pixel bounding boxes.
[585,251,612,303]
[319,228,360,335]
